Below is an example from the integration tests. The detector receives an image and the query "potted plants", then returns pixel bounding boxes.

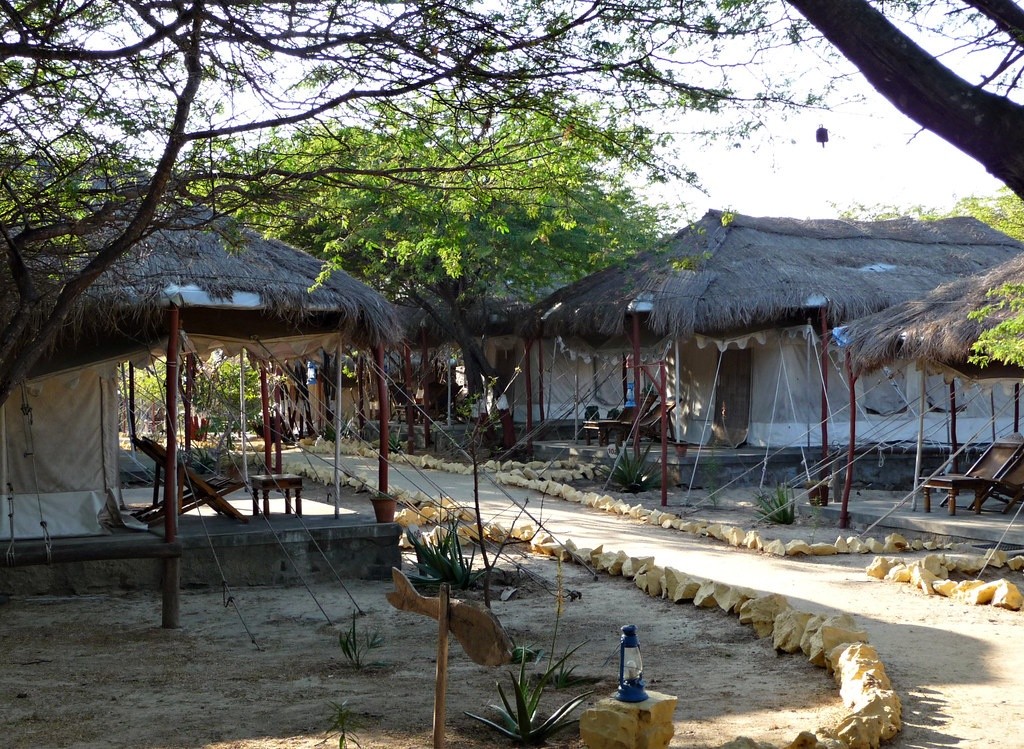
[674,440,691,457]
[369,489,400,523]
[804,480,829,507]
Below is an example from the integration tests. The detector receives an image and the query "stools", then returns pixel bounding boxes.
[249,473,303,519]
[582,420,622,448]
[395,404,424,425]
[918,475,985,516]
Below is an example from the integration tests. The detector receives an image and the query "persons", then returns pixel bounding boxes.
[415,382,424,404]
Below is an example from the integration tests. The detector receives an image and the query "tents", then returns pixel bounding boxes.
[0,209,1024,626]
